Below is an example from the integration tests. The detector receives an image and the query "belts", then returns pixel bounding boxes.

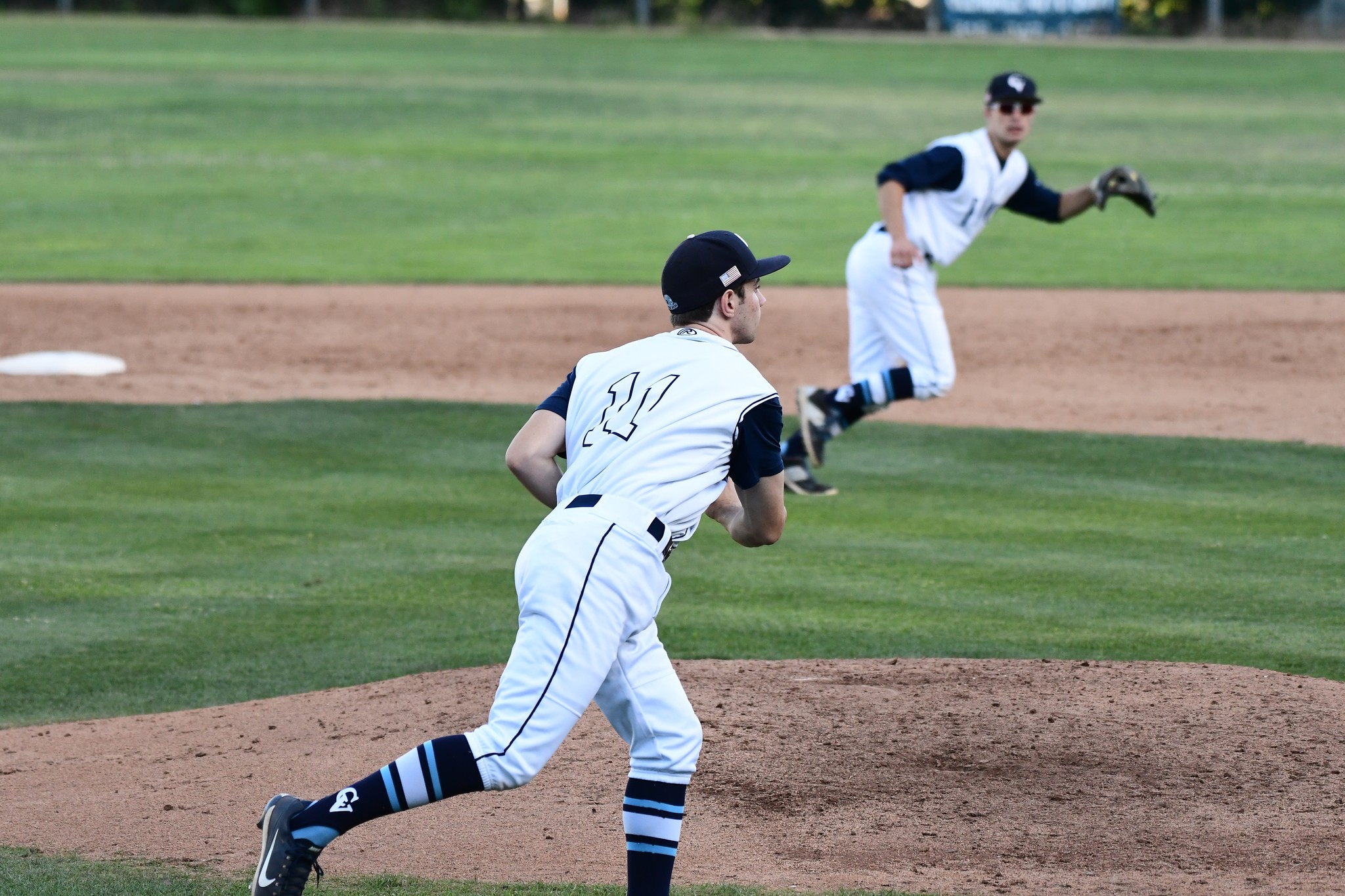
[879,225,933,266]
[565,497,674,562]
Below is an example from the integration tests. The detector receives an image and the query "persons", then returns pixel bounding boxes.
[779,73,1156,497]
[248,229,790,896]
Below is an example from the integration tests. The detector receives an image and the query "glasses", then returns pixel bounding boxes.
[990,101,1033,115]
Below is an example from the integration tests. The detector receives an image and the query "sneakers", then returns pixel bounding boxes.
[252,791,326,896]
[797,386,833,467]
[777,441,839,496]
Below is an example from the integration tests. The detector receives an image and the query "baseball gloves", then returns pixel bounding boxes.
[1090,165,1156,219]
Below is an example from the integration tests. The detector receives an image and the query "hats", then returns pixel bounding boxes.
[990,73,1040,106]
[662,230,792,317]
